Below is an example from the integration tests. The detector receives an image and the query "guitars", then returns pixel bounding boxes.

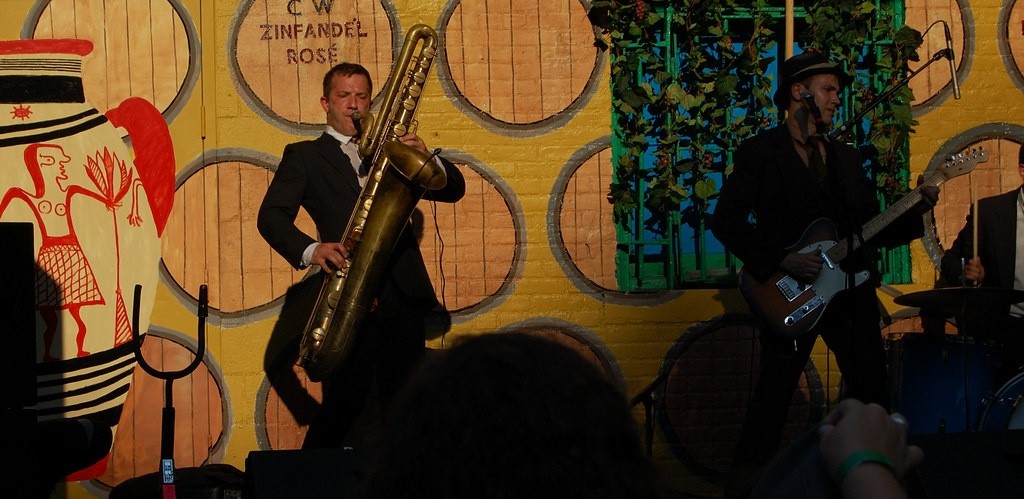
[741,144,988,340]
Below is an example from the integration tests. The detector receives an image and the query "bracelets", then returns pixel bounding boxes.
[835,450,895,484]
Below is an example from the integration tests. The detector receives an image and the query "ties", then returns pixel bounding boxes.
[807,137,826,184]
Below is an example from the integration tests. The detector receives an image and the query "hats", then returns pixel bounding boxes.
[774,51,852,109]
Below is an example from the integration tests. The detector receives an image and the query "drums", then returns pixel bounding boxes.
[883,331,1004,436]
[977,369,1024,434]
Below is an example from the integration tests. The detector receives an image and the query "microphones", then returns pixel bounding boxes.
[409,147,442,192]
[944,25,962,100]
[800,89,828,135]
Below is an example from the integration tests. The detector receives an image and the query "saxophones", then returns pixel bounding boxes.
[293,24,449,376]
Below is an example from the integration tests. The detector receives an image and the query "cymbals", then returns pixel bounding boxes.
[893,284,1024,309]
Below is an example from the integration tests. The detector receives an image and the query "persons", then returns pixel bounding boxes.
[258,63,466,449]
[710,52,939,499]
[940,140,1024,397]
[818,398,923,499]
